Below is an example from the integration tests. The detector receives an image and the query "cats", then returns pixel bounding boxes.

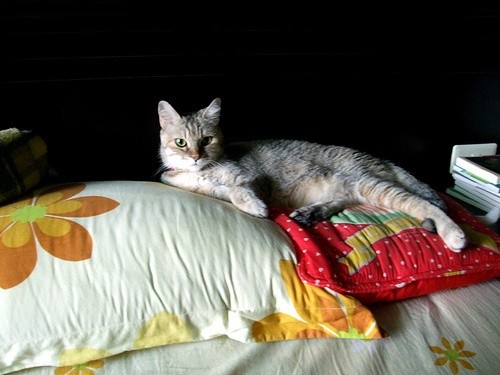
[151,98,467,254]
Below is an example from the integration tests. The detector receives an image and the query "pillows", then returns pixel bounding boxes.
[268,189,500,309]
[0,178,389,375]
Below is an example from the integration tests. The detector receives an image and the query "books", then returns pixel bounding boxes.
[447,156,500,217]
